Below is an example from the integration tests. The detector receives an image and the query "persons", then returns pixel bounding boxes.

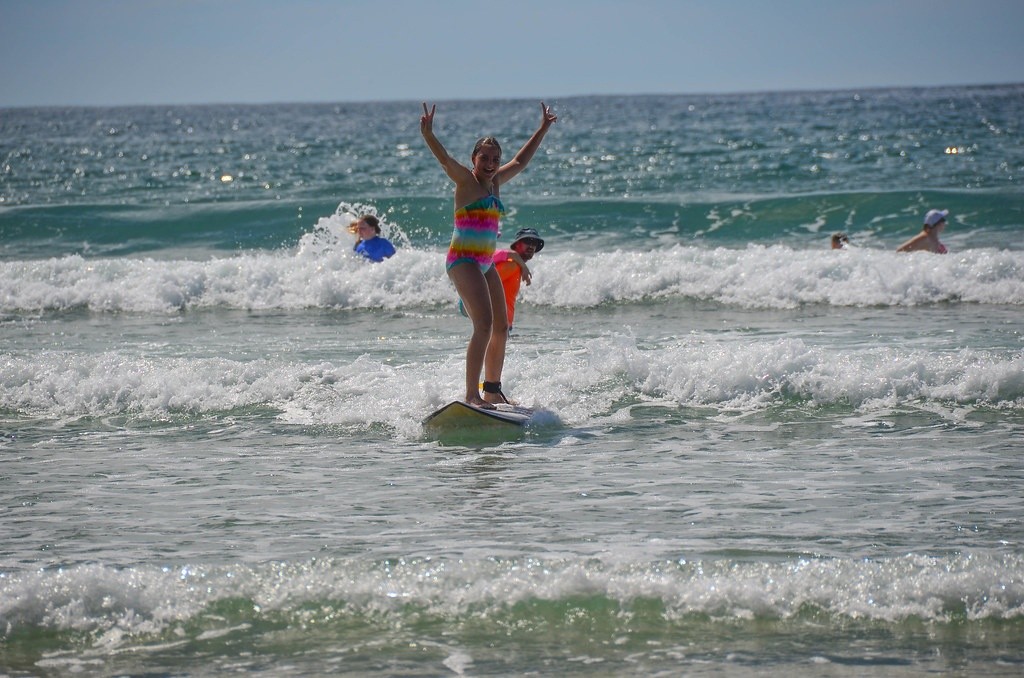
[346,215,396,262]
[493,227,544,330]
[831,232,849,249]
[419,101,558,409]
[896,209,949,254]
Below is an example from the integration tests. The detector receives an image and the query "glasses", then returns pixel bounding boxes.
[524,238,538,246]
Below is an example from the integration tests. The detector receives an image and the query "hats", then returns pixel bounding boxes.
[510,227,544,254]
[925,208,948,228]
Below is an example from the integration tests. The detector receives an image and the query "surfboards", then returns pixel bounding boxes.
[422,400,537,427]
